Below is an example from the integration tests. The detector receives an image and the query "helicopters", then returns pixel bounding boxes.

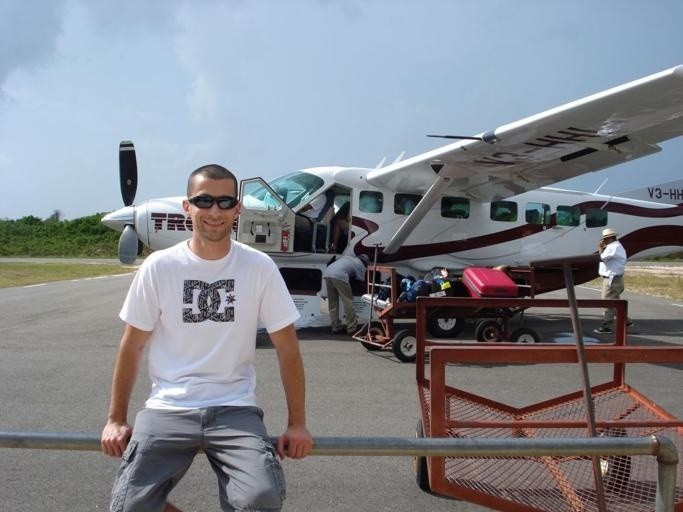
[102,64,683,328]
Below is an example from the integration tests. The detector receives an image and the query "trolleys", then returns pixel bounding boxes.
[352,265,541,363]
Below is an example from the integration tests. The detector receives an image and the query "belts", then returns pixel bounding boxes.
[602,275,623,278]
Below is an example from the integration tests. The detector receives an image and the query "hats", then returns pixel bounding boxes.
[359,253,370,265]
[602,229,619,239]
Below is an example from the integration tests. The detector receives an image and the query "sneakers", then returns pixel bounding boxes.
[594,327,613,334]
[625,322,635,329]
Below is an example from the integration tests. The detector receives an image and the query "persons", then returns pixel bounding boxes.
[327,202,351,254]
[322,252,371,338]
[101,165,313,512]
[591,228,635,334]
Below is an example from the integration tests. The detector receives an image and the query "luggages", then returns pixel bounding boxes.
[462,268,518,298]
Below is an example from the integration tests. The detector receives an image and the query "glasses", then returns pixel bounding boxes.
[186,195,237,210]
